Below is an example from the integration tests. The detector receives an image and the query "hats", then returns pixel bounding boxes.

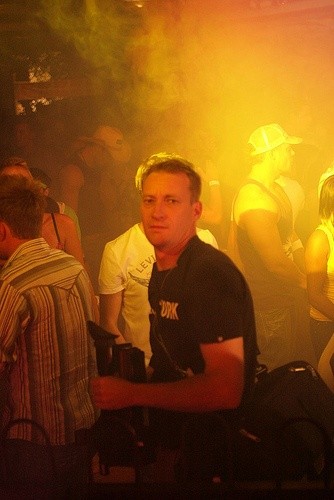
[248,124,303,156]
[30,165,53,190]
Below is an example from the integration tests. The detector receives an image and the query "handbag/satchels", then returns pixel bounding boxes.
[237,351,334,500]
[1,418,90,499]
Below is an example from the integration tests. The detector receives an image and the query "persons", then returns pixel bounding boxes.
[0,110,334,500]
